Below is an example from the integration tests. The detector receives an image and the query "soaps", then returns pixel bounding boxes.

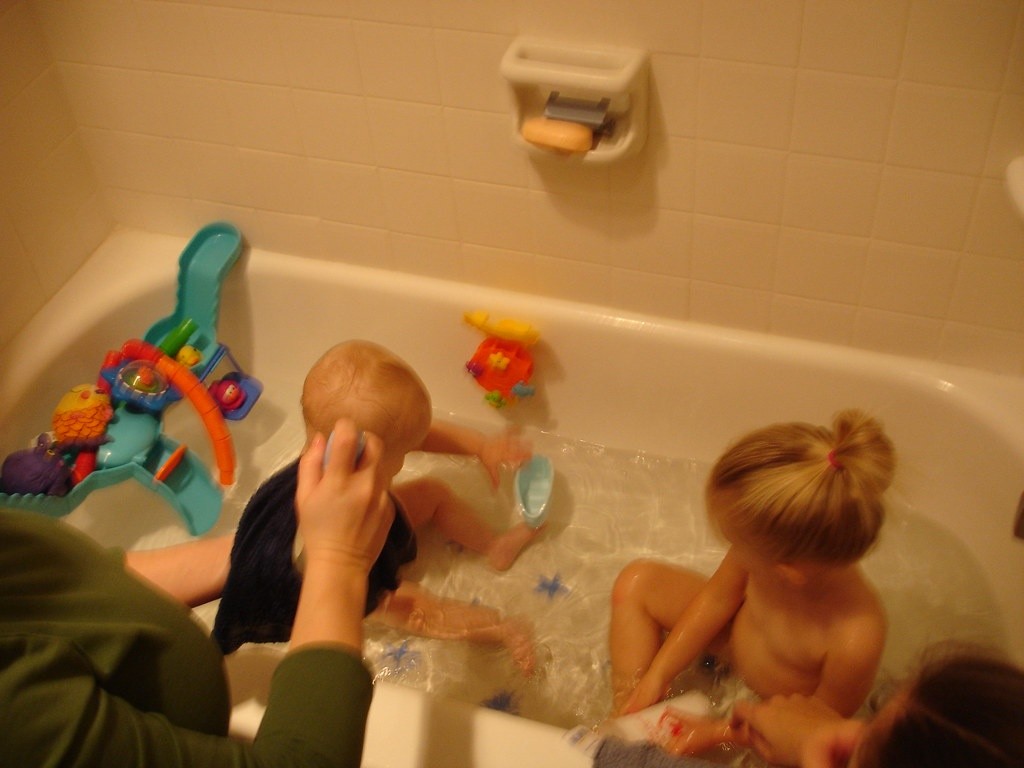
[519,113,595,160]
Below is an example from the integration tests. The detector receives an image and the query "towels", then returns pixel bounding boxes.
[212,456,420,655]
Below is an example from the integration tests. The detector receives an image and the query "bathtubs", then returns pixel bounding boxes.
[1,225,1024,768]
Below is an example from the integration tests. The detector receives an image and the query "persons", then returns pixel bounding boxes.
[609,408,895,715]
[214,339,543,676]
[0,417,396,768]
[730,641,1024,768]
